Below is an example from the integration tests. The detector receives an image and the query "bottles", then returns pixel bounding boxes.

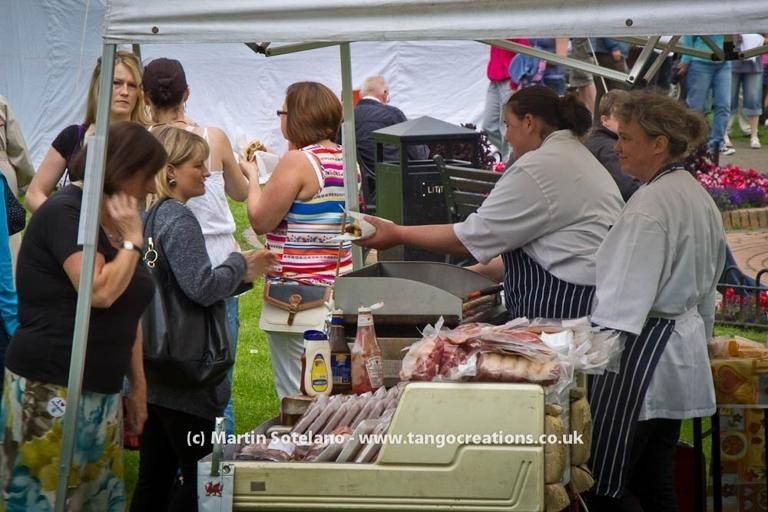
[302,328,333,398]
[351,307,385,392]
[329,312,354,393]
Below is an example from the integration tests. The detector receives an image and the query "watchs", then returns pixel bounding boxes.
[120,240,141,252]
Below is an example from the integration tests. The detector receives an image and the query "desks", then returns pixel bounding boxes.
[690,398,767,512]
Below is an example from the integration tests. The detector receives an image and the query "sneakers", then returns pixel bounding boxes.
[750,137,761,149]
[720,145,737,156]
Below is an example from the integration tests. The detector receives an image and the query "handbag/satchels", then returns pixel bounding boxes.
[257,283,333,335]
[137,196,238,393]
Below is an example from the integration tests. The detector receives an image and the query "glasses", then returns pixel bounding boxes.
[276,110,288,118]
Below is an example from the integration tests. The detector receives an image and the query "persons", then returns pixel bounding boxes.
[2,180,33,499]
[24,49,148,450]
[141,57,249,456]
[2,122,169,510]
[1,95,37,286]
[241,82,365,430]
[531,91,728,512]
[480,33,768,161]
[129,125,271,512]
[337,75,409,208]
[350,85,626,323]
[586,88,641,203]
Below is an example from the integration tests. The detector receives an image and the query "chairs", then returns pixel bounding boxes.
[430,153,510,263]
[353,150,380,269]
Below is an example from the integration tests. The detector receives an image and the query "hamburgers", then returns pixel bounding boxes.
[245,140,265,161]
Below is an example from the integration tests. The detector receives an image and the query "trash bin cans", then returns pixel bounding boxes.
[369,115,482,263]
